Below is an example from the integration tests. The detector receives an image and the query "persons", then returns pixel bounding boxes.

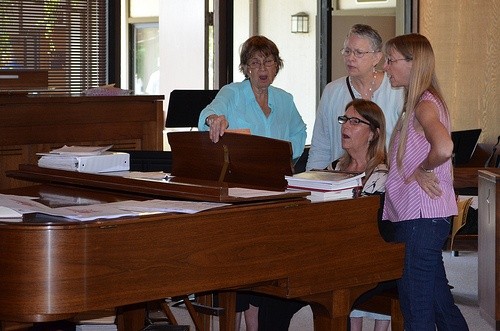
[306,23,406,171]
[379,33,471,330]
[197,36,308,170]
[325,99,390,199]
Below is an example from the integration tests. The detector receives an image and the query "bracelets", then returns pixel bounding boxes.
[419,163,434,173]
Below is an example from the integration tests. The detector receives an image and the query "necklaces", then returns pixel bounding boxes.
[349,72,377,96]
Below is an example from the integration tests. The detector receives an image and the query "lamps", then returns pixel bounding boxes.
[291,12,310,36]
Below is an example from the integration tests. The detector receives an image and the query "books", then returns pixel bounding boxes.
[285,170,366,203]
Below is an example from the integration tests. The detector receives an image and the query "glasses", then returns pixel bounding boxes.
[386,58,409,65]
[338,116,376,130]
[340,48,382,57]
[245,59,276,68]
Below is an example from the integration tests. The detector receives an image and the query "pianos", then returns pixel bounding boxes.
[0,126,411,331]
